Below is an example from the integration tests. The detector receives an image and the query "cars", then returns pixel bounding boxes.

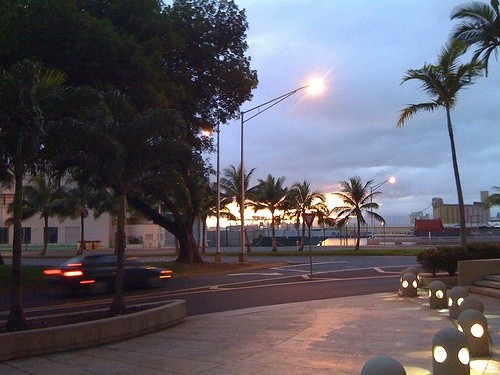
[42,251,173,298]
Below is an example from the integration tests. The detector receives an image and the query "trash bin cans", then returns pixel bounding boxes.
[93,241,102,249]
[84,241,93,250]
[77,241,84,250]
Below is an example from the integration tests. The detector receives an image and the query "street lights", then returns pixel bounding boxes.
[237,77,327,265]
[369,176,396,238]
[201,121,221,262]
